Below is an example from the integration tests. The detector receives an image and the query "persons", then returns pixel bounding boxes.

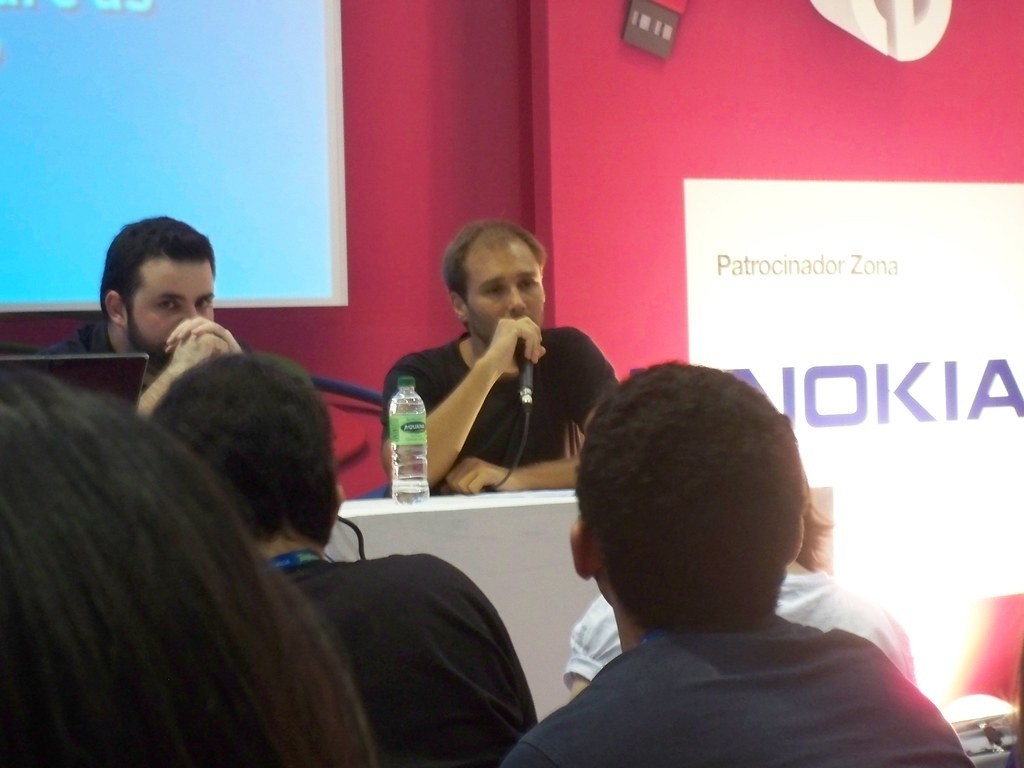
[382,221,619,494]
[502,362,979,767]
[0,218,538,768]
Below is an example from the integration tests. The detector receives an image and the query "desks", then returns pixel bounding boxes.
[325,483,833,722]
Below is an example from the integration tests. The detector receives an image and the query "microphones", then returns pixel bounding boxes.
[516,343,534,412]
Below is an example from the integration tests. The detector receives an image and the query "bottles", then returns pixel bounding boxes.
[387,375,429,509]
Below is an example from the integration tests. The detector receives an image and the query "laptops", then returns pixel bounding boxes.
[0,353,149,412]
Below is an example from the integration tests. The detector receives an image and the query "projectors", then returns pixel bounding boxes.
[941,694,1019,736]
